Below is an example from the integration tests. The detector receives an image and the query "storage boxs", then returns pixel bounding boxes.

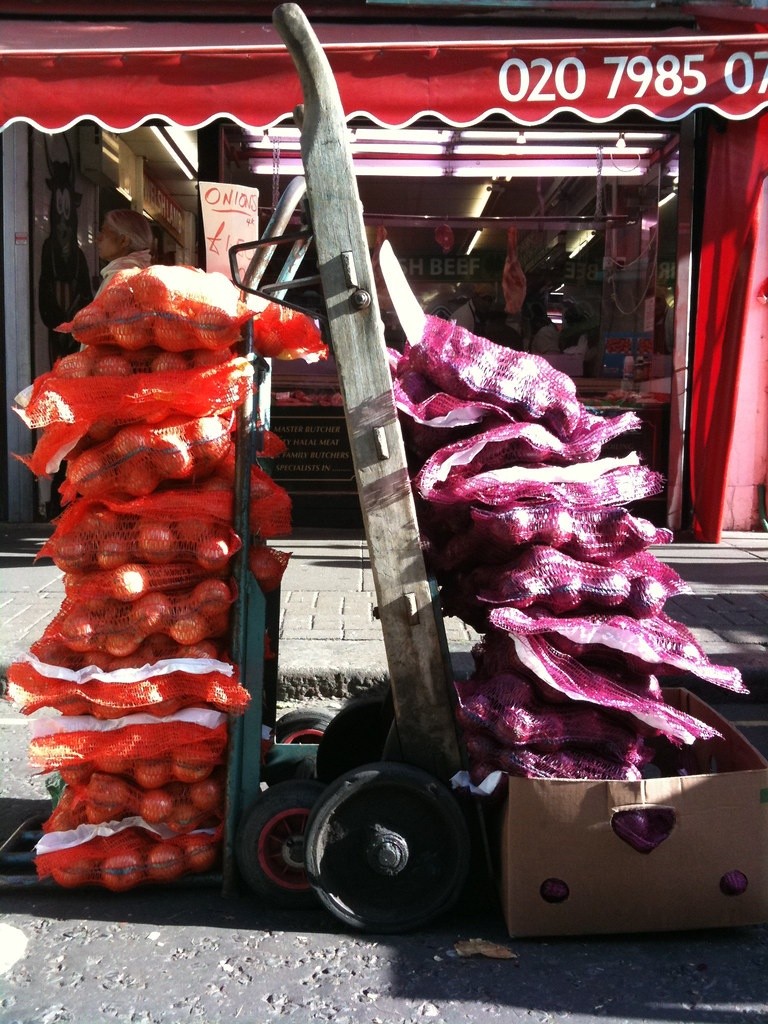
[636,334,653,362]
[541,354,584,377]
[495,686,768,939]
[596,333,636,378]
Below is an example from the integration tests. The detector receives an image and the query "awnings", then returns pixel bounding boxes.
[0,0,768,140]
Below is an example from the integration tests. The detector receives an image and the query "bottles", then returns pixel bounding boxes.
[623,351,634,381]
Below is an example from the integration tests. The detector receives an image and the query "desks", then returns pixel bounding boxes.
[572,377,635,393]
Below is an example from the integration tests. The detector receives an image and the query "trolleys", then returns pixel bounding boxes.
[0,2,489,943]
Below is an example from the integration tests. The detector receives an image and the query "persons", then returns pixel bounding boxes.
[427,283,605,378]
[653,286,673,379]
[80,210,152,350]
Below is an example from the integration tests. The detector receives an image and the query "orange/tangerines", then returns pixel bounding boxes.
[5,262,324,887]
